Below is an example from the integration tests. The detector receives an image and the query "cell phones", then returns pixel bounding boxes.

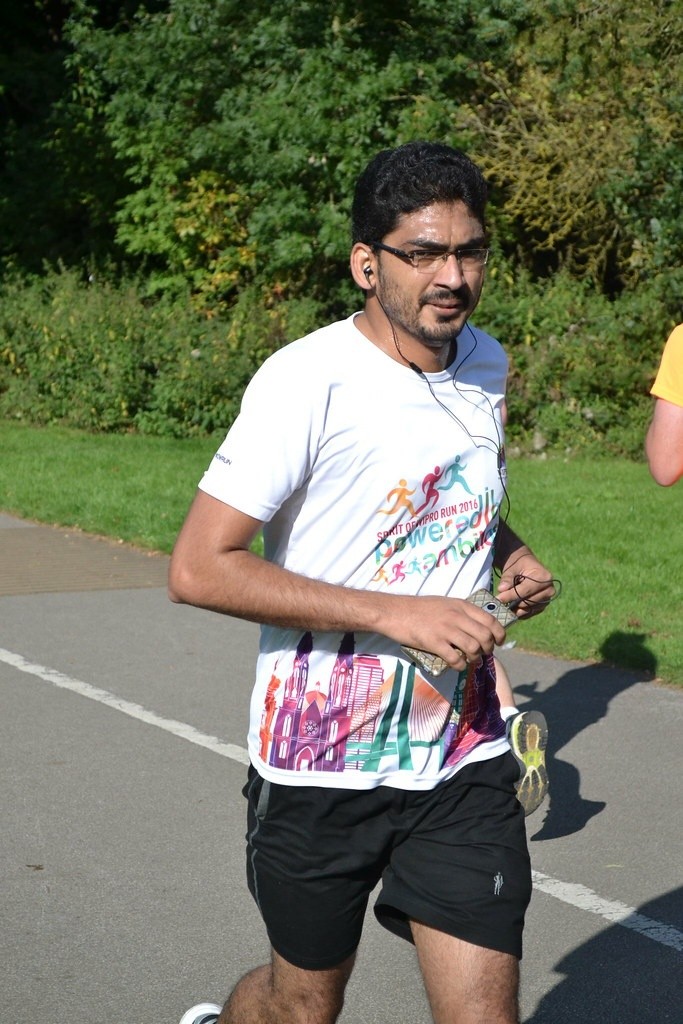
[400,588,518,678]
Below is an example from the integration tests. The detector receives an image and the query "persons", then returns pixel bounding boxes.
[493,657,548,818]
[646,323,683,487]
[169,141,555,1024]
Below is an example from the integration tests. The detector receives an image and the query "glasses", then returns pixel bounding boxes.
[370,240,490,273]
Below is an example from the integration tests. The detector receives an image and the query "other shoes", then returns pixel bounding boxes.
[179,1001,224,1024]
[505,710,551,817]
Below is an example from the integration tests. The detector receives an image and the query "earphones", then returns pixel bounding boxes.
[364,265,374,276]
